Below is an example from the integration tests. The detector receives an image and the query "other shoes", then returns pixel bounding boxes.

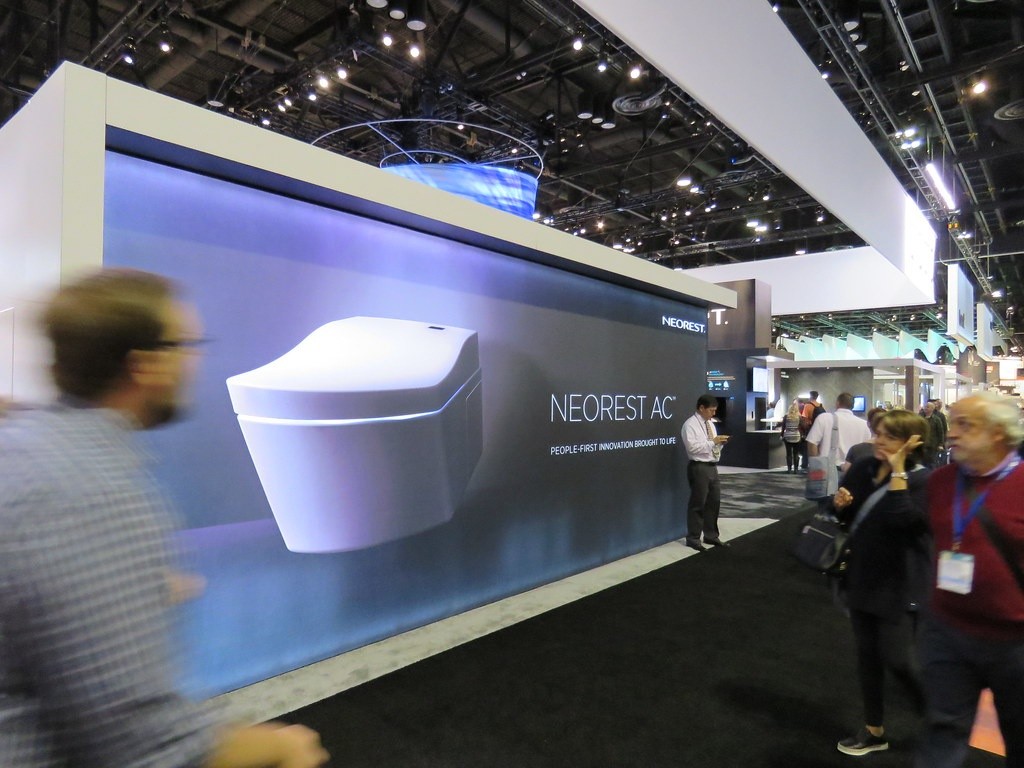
[794,469,798,473]
[788,470,791,473]
[801,469,808,474]
[837,727,889,756]
[686,542,708,553]
[703,537,730,548]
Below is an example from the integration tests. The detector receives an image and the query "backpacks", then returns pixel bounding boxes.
[808,402,826,425]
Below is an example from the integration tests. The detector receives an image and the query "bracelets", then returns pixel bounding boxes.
[891,471,910,480]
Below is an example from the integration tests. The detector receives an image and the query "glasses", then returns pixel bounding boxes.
[116,335,213,370]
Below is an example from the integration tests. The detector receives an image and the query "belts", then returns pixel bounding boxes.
[688,460,717,466]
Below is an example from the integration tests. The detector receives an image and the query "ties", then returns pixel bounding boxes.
[705,421,720,458]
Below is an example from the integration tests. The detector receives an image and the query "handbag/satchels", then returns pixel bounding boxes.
[788,497,846,571]
[806,456,838,499]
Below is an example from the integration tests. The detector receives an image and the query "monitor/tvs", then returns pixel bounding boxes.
[851,396,865,412]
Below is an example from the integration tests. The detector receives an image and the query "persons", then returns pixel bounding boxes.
[840,408,887,471]
[680,395,731,552]
[2,269,330,768]
[766,401,776,420]
[779,391,823,474]
[922,402,944,465]
[805,392,1024,768]
